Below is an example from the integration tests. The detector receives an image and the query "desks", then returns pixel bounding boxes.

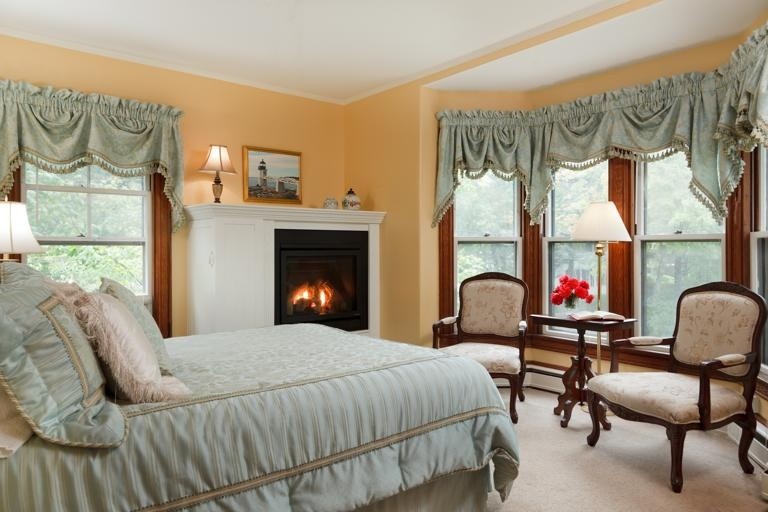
[528,314,639,431]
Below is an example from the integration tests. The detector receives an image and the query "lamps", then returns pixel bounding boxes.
[572,200,632,418]
[197,144,237,204]
[0,193,46,263]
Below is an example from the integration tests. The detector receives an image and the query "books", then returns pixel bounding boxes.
[568,309,626,324]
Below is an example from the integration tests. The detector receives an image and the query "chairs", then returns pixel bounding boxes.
[585,281,768,494]
[432,272,530,423]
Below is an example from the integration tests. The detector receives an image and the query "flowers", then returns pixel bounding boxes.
[550,274,594,311]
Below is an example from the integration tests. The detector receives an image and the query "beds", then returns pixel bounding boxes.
[1,321,496,512]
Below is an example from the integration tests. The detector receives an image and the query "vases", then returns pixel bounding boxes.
[565,304,575,316]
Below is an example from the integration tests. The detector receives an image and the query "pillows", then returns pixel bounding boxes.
[0,256,176,450]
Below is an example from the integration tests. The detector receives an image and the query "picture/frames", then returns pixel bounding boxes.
[242,145,303,204]
